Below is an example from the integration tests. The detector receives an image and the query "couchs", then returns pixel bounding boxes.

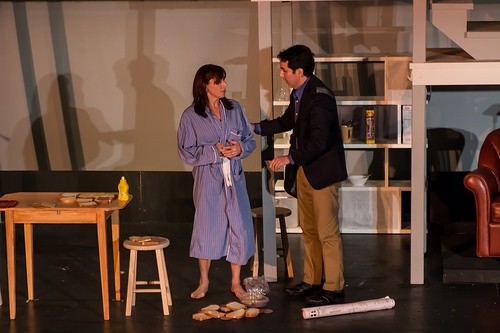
[463,128,500,257]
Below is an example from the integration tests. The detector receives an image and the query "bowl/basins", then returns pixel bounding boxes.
[348,174,369,186]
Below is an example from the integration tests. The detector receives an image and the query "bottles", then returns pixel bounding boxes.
[118,176,129,201]
[278,89,285,101]
[365,110,376,144]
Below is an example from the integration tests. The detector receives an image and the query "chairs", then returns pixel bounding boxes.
[425,128,474,227]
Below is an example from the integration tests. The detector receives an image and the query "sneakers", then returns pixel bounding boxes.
[286,282,322,297]
[305,290,344,306]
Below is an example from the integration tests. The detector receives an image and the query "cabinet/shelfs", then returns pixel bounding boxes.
[271,51,413,234]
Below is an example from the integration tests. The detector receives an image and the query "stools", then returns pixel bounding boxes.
[123,236,172,317]
[249,207,294,279]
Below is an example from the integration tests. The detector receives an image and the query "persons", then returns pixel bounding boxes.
[246,44,347,305]
[177,64,257,301]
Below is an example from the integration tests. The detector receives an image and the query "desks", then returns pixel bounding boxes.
[0,192,133,321]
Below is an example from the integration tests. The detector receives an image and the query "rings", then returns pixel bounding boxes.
[232,153,234,155]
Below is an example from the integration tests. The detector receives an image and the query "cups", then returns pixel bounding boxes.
[341,126,353,144]
